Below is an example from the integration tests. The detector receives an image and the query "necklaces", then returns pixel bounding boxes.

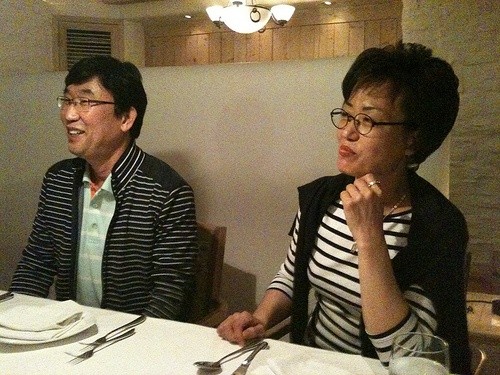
[350,194,407,253]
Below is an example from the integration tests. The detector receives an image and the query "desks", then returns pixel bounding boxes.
[0,291,391,375]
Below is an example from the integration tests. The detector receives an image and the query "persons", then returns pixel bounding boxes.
[7,55,196,320]
[216,43,472,375]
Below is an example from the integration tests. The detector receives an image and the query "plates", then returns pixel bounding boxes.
[0,304,95,345]
[250,363,347,375]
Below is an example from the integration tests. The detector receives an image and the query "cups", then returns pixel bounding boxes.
[389,333,450,375]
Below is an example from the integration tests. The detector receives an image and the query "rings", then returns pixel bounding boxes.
[368,180,380,188]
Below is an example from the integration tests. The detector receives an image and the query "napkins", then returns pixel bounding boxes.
[0,299,83,342]
[265,349,372,375]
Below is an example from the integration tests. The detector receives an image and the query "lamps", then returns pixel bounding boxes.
[206,0,295,34]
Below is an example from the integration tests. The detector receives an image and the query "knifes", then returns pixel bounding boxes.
[231,342,268,375]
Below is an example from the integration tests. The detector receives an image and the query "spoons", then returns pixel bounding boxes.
[193,341,262,370]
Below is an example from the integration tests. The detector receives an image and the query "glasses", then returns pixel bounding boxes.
[57,96,117,113]
[331,108,405,136]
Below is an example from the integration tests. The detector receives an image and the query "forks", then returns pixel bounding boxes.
[64,328,136,358]
[79,314,146,345]
